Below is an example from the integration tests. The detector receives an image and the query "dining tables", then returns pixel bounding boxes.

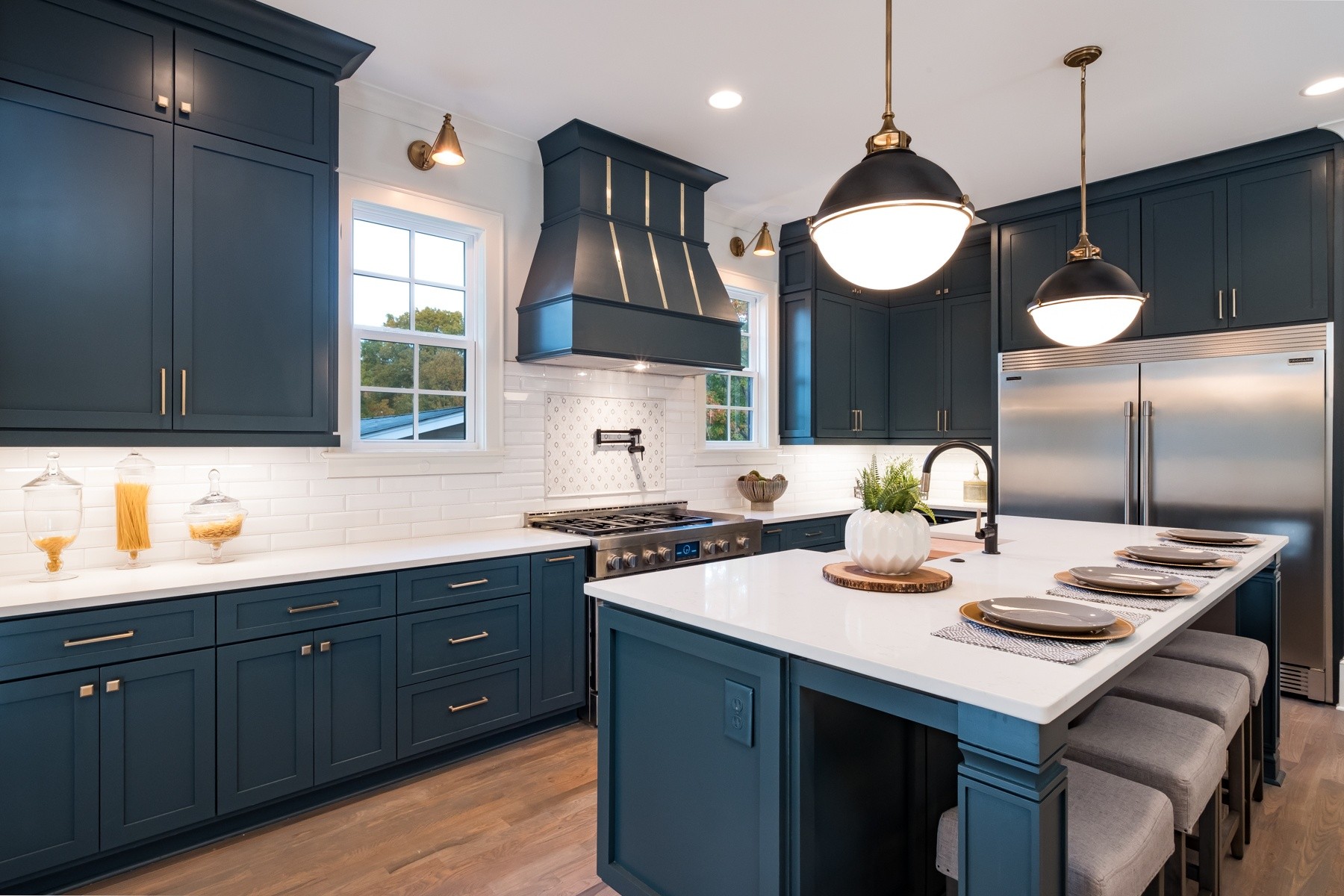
[582,511,1296,896]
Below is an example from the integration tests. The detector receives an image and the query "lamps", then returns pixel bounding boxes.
[729,221,777,258]
[1022,44,1152,348]
[406,113,466,173]
[805,1,977,291]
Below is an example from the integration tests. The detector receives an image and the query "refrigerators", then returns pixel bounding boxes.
[996,321,1344,707]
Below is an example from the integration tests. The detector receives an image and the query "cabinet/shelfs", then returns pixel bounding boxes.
[0,0,378,453]
[0,526,600,895]
[989,318,1344,706]
[773,126,1344,450]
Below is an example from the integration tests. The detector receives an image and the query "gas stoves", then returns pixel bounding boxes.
[526,498,763,577]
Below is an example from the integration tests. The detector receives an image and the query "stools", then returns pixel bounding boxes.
[1060,694,1235,895]
[933,754,1180,895]
[1111,655,1255,861]
[1150,629,1272,846]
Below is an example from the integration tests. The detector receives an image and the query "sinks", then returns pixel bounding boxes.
[831,532,1016,569]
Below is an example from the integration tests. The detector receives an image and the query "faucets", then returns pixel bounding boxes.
[923,441,1000,556]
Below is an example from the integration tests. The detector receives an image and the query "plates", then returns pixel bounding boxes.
[959,530,1264,639]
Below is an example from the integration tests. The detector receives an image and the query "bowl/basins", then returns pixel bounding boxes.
[737,479,789,512]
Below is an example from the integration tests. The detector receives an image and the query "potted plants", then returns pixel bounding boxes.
[843,451,939,577]
